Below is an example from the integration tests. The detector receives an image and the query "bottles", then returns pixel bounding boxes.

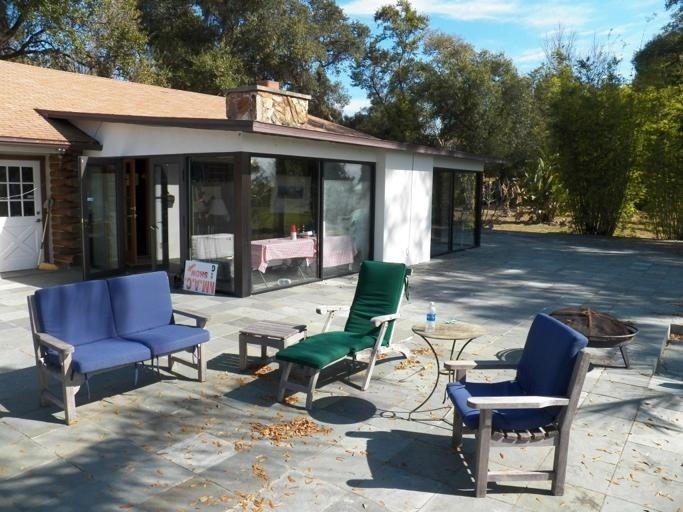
[425,302,437,332]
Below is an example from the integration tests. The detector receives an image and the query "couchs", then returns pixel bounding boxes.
[24,268,212,425]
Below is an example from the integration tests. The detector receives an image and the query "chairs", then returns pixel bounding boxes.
[273,255,411,414]
[444,310,592,503]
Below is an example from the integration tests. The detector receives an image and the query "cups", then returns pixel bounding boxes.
[290,232,297,240]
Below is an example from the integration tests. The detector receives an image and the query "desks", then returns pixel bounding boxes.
[249,233,354,288]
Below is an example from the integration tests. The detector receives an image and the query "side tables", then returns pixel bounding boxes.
[406,321,480,428]
[237,320,307,372]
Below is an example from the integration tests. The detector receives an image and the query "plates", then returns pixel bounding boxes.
[297,235,313,237]
